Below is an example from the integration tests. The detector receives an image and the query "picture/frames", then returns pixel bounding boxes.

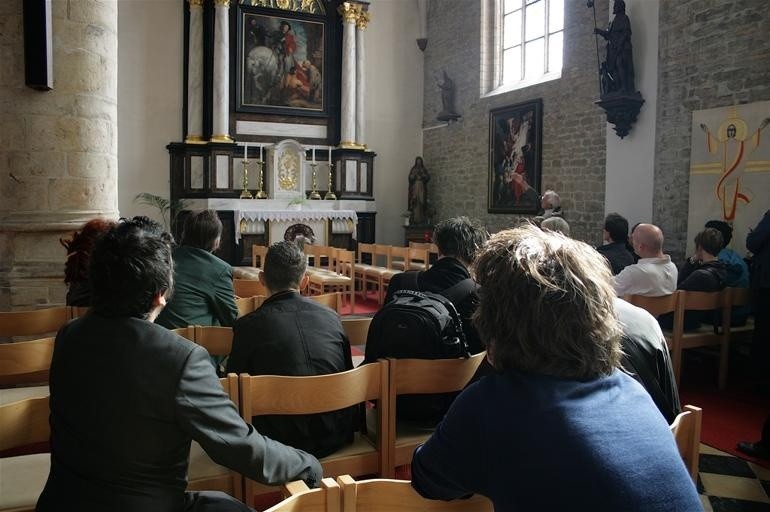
[232,4,333,118]
[487,98,542,214]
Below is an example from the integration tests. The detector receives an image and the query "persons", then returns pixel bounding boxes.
[541,190,565,219]
[586,0,635,97]
[226,241,360,458]
[611,224,680,298]
[596,213,634,275]
[678,227,726,334]
[245,14,323,108]
[407,156,431,228]
[63,218,115,306]
[699,116,770,220]
[153,210,241,330]
[383,217,486,426]
[438,70,457,117]
[540,216,570,236]
[411,225,705,512]
[736,211,770,460]
[706,220,750,327]
[612,297,682,425]
[35,216,323,512]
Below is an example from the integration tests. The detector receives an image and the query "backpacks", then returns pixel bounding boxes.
[366,270,482,360]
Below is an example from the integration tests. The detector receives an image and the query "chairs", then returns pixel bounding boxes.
[388,350,488,483]
[341,242,375,302]
[672,408,705,494]
[308,250,355,316]
[305,243,333,272]
[0,338,54,385]
[0,307,71,341]
[679,288,728,398]
[250,469,343,510]
[239,359,387,507]
[380,246,410,305]
[180,373,242,503]
[195,325,237,357]
[252,244,268,271]
[340,467,491,512]
[391,240,427,272]
[293,289,342,318]
[233,278,270,297]
[0,394,55,511]
[426,243,438,272]
[363,245,391,306]
[730,287,748,333]
[619,289,678,397]
[340,321,376,349]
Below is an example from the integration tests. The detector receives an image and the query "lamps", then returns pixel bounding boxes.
[416,0,427,51]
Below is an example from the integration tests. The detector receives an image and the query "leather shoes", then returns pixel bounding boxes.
[736,440,770,461]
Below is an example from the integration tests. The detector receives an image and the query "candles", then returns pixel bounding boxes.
[329,145,331,165]
[244,142,247,161]
[312,146,316,163]
[260,143,263,162]
